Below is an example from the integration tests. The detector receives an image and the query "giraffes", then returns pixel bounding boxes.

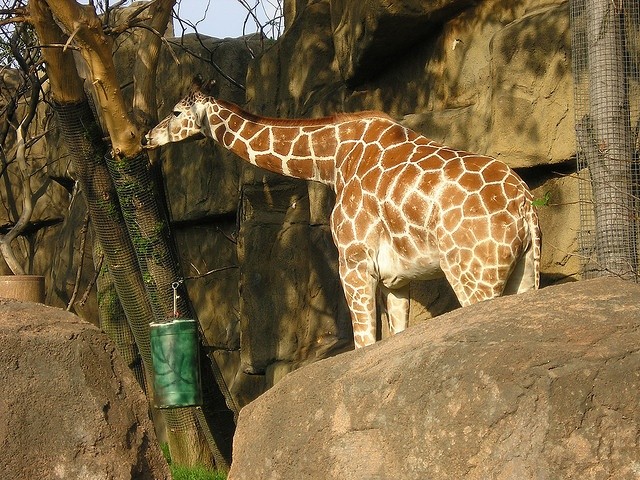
[141,73,540,351]
